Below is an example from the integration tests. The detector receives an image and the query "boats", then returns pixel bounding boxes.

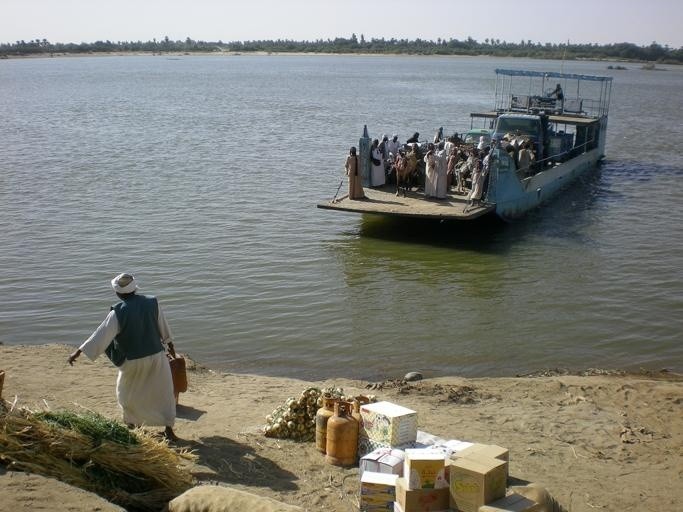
[315,66,614,228]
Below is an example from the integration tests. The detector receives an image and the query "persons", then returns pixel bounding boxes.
[370,127,536,206]
[345,146,364,200]
[551,84,563,99]
[66,273,181,440]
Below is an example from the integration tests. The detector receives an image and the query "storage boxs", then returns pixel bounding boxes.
[354,399,554,512]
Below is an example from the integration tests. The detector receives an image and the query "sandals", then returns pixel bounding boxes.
[159,431,177,442]
